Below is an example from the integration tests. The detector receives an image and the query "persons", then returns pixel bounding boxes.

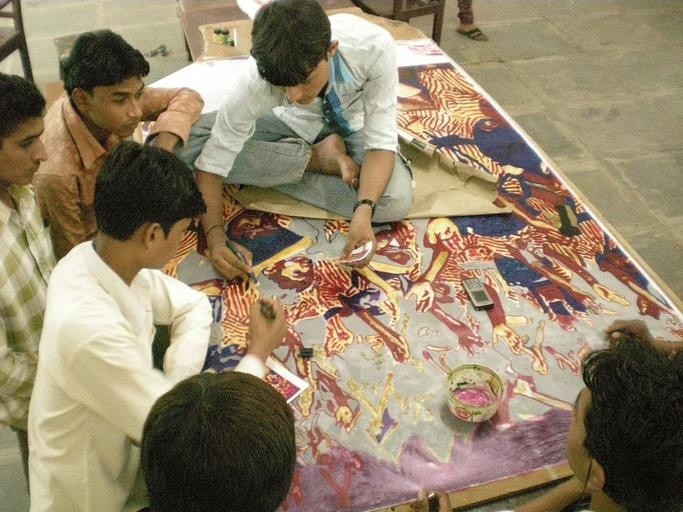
[507,319,683,512]
[136,369,295,512]
[25,141,288,512]
[456,0,489,41]
[170,1,416,279]
[0,72,61,484]
[30,28,205,260]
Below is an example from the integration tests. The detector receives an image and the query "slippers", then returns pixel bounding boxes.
[456,27,488,42]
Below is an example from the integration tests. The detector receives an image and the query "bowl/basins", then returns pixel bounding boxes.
[445,364,505,423]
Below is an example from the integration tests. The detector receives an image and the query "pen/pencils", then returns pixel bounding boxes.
[261,303,276,320]
[226,240,255,280]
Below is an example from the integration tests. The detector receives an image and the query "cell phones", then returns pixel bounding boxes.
[461,277,494,309]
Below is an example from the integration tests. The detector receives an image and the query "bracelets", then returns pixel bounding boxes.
[207,224,225,236]
[352,199,377,218]
